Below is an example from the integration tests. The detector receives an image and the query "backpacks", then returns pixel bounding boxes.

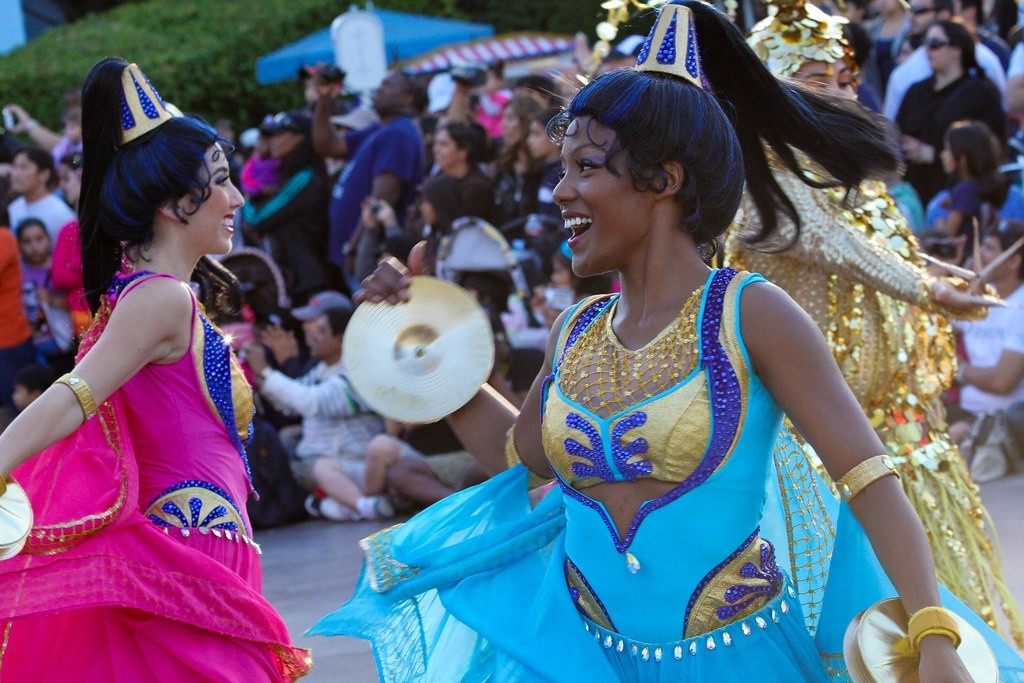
[245,420,300,530]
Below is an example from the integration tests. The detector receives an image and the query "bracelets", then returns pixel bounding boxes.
[908,606,962,652]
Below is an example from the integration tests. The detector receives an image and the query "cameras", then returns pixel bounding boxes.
[321,65,345,82]
[370,202,380,212]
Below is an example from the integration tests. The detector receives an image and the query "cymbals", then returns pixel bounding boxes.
[841,596,1001,682]
[0,471,34,563]
[340,274,497,427]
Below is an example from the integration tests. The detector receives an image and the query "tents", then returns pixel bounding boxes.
[257,2,492,85]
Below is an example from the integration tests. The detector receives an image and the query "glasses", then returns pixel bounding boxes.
[997,220,1021,242]
[926,39,957,49]
[273,112,299,132]
[912,3,940,16]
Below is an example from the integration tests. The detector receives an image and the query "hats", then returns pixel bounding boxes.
[258,109,314,136]
[289,294,352,324]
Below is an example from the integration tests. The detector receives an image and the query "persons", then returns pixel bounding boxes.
[0,62,624,523]
[304,1,1024,683]
[711,1,1024,654]
[825,0,1024,483]
[0,58,312,683]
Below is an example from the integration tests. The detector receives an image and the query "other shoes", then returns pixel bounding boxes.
[971,448,1008,482]
[370,493,395,518]
[305,494,357,522]
